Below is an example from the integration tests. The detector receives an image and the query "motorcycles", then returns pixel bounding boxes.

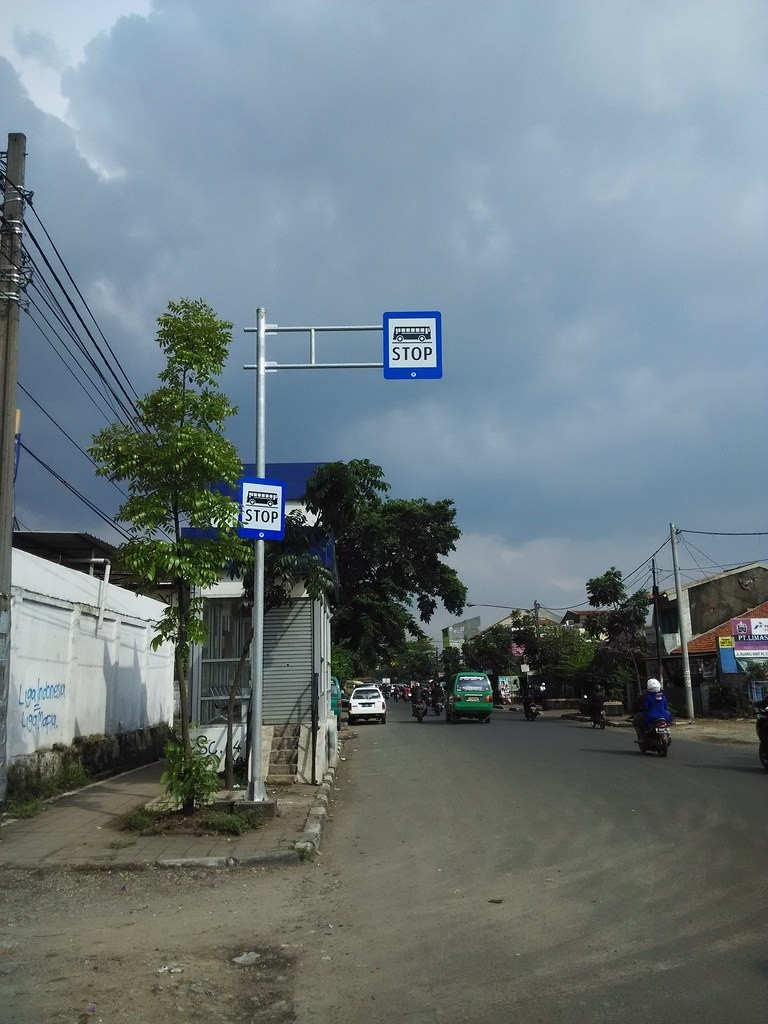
[584,694,606,729]
[628,714,671,757]
[413,699,426,723]
[434,696,444,716]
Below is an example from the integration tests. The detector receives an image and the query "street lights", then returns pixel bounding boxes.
[466,603,541,642]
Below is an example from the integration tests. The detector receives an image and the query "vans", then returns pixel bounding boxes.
[447,672,493,723]
[331,677,344,731]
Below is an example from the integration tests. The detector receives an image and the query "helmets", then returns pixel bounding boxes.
[594,684,601,691]
[646,678,661,692]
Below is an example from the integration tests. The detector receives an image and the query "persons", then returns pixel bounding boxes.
[380,680,445,713]
[632,677,673,745]
[520,682,548,709]
[589,684,605,722]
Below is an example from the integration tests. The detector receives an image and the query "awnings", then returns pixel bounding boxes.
[736,658,768,673]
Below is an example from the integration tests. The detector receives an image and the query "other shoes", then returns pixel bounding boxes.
[641,730,649,737]
[634,739,646,744]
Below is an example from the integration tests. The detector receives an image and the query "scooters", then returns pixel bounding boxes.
[524,703,539,720]
[393,693,433,708]
[756,707,768,768]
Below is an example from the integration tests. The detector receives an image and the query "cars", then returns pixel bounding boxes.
[348,687,388,724]
